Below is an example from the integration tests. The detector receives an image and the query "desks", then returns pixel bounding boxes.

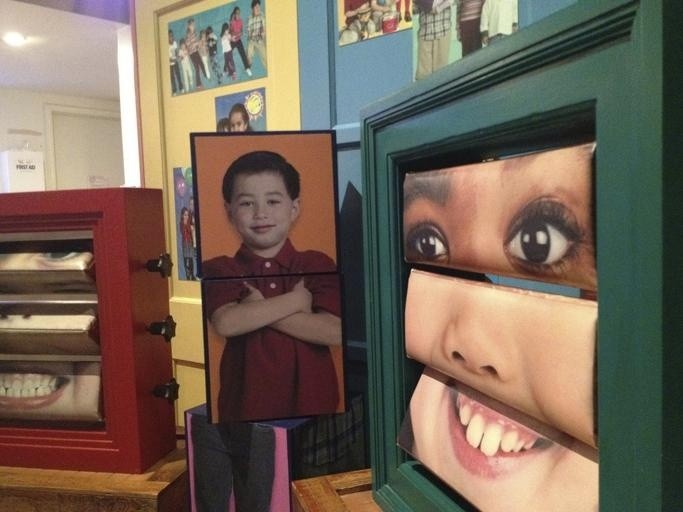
[291,469,384,512]
[0,428,191,512]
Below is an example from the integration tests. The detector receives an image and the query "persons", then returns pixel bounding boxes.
[202,148,343,425]
[398,141,595,290]
[188,196,201,280]
[398,366,599,510]
[217,118,229,133]
[188,415,277,512]
[164,1,266,96]
[0,251,90,269]
[339,1,518,82]
[227,103,253,132]
[178,207,196,281]
[0,310,96,332]
[0,371,102,417]
[403,268,596,448]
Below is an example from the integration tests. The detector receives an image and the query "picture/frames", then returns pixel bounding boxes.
[360,0,683,512]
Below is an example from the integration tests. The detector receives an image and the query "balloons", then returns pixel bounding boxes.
[175,174,187,199]
[184,168,193,188]
